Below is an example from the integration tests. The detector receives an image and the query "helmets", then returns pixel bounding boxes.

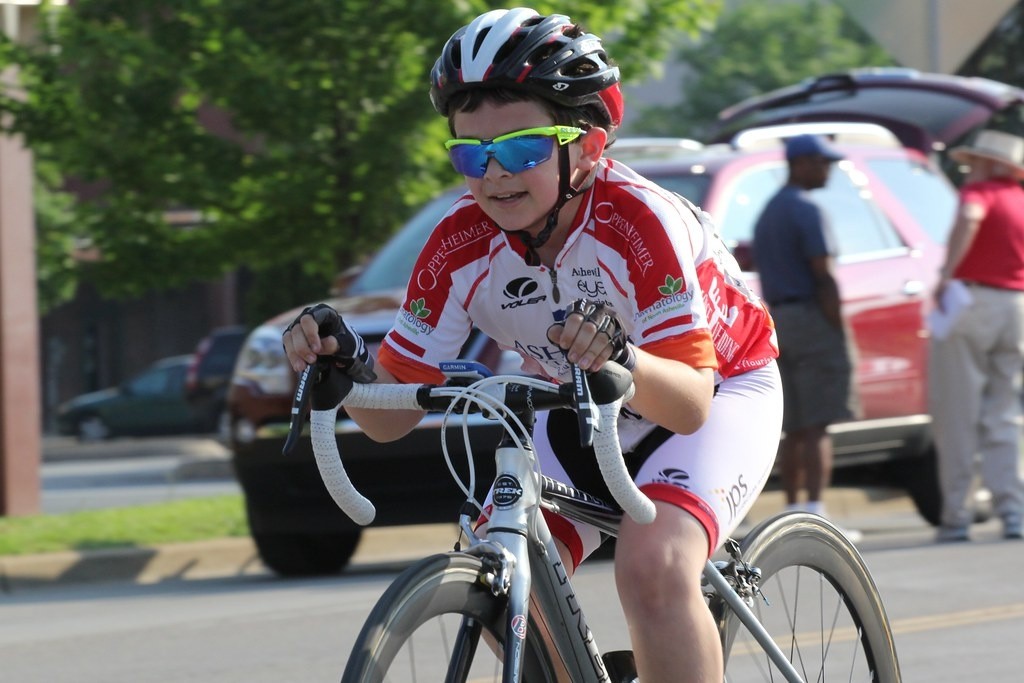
[425,6,625,267]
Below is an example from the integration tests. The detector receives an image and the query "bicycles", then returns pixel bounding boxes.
[278,324,903,683]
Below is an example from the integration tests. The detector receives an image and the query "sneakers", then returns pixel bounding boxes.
[997,513,1022,536]
[782,502,864,546]
[932,522,972,541]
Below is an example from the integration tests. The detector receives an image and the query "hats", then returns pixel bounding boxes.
[947,129,1023,172]
[787,135,845,161]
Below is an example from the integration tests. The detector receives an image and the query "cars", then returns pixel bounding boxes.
[183,319,250,433]
[227,63,1023,577]
[59,352,225,442]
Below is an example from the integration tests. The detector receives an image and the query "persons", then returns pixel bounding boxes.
[926,129,1024,542]
[754,134,863,544]
[282,7,783,683]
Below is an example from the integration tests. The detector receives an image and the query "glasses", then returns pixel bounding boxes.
[445,125,590,176]
